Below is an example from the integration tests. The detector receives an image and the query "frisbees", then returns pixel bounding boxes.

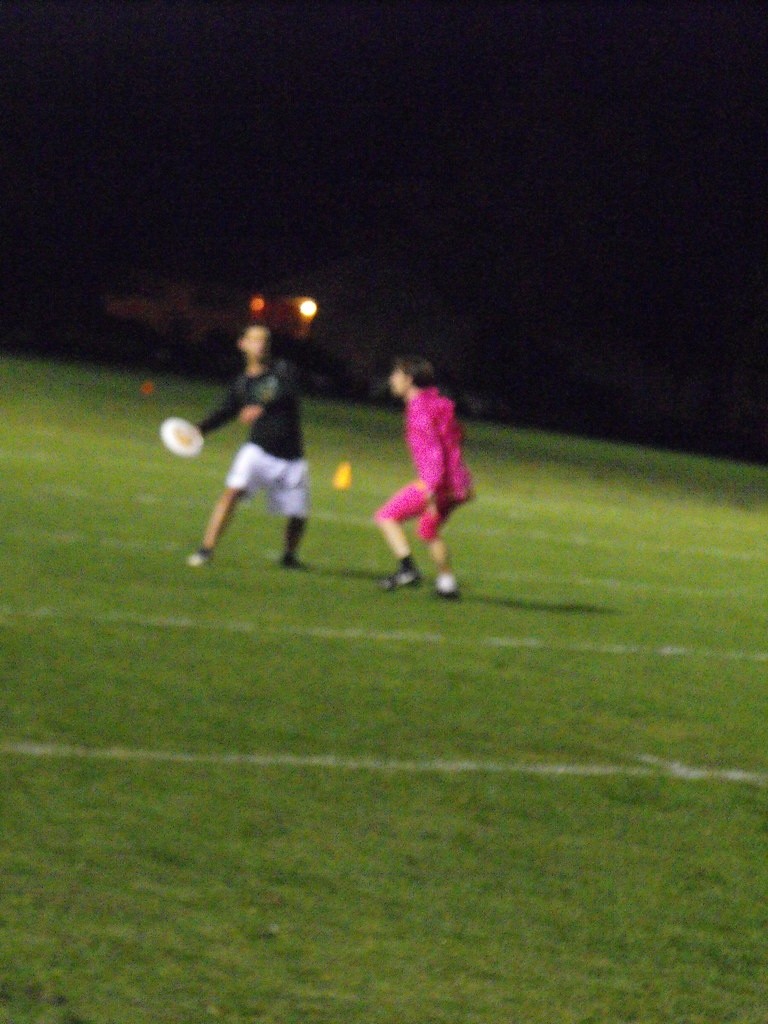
[161,414,203,457]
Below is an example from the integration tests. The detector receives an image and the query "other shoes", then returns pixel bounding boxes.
[379,563,422,592]
[186,547,214,565]
[280,552,306,570]
[436,582,460,602]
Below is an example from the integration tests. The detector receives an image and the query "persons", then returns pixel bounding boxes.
[374,350,473,603]
[186,320,312,572]
